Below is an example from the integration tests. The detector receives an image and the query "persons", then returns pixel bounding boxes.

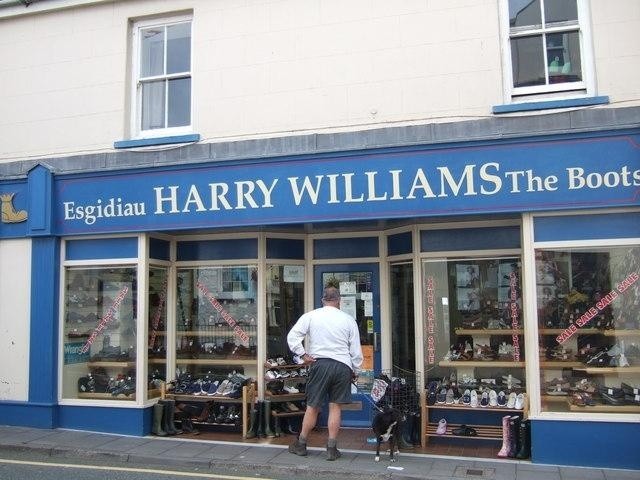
[285,282,364,461]
[466,265,480,309]
[503,263,521,301]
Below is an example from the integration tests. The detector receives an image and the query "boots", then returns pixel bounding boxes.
[498,416,531,459]
[398,412,420,450]
[152,399,199,435]
[247,400,275,438]
[275,417,298,436]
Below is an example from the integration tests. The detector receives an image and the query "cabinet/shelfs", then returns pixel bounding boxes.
[419,328,639,448]
[67,262,315,433]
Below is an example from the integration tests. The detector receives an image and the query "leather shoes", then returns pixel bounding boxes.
[546,378,640,405]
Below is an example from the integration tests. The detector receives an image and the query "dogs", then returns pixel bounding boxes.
[372,407,406,463]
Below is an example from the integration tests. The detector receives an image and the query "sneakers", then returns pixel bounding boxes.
[265,356,308,413]
[436,419,447,435]
[197,401,243,423]
[66,275,136,337]
[453,425,475,436]
[327,445,341,460]
[168,372,250,397]
[445,341,515,361]
[545,343,640,367]
[289,439,306,456]
[426,372,525,409]
